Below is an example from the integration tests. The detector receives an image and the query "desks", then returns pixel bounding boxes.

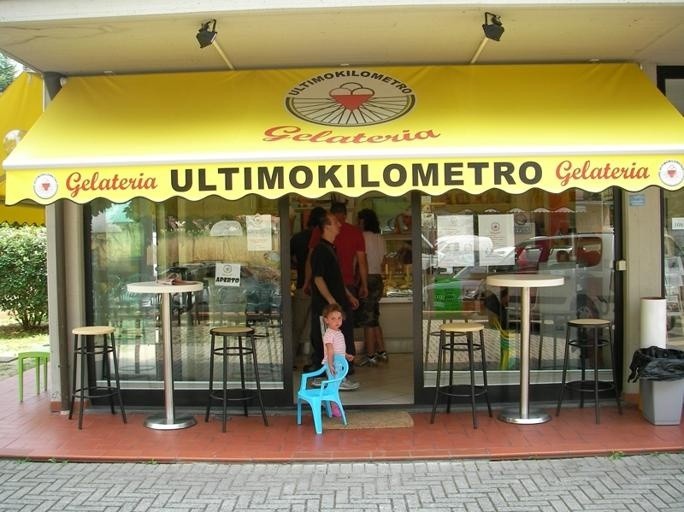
[486,274,565,424]
[126,280,204,430]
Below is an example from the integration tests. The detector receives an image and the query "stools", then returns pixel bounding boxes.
[69,326,127,430]
[206,326,270,434]
[430,322,493,430]
[558,318,624,424]
[15,348,50,403]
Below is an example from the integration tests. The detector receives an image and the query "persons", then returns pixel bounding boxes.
[288,201,390,390]
[320,302,356,419]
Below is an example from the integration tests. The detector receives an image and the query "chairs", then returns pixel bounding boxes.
[507,260,577,370]
[92,262,281,381]
[297,353,349,434]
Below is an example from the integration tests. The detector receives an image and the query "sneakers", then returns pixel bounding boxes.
[374,352,388,363]
[356,356,379,368]
[303,365,360,391]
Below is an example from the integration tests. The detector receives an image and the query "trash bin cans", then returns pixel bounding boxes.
[628,348,684,426]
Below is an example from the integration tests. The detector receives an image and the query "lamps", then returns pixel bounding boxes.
[207,219,243,262]
[482,13,504,42]
[196,18,217,48]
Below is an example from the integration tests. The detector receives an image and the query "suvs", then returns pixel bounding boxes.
[478,232,684,336]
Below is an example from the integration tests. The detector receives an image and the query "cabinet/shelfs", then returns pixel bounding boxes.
[291,233,412,354]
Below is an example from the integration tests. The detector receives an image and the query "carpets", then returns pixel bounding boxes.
[320,409,415,429]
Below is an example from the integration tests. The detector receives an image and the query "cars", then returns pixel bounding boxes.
[110,260,282,317]
[383,287,413,299]
[427,247,504,310]
